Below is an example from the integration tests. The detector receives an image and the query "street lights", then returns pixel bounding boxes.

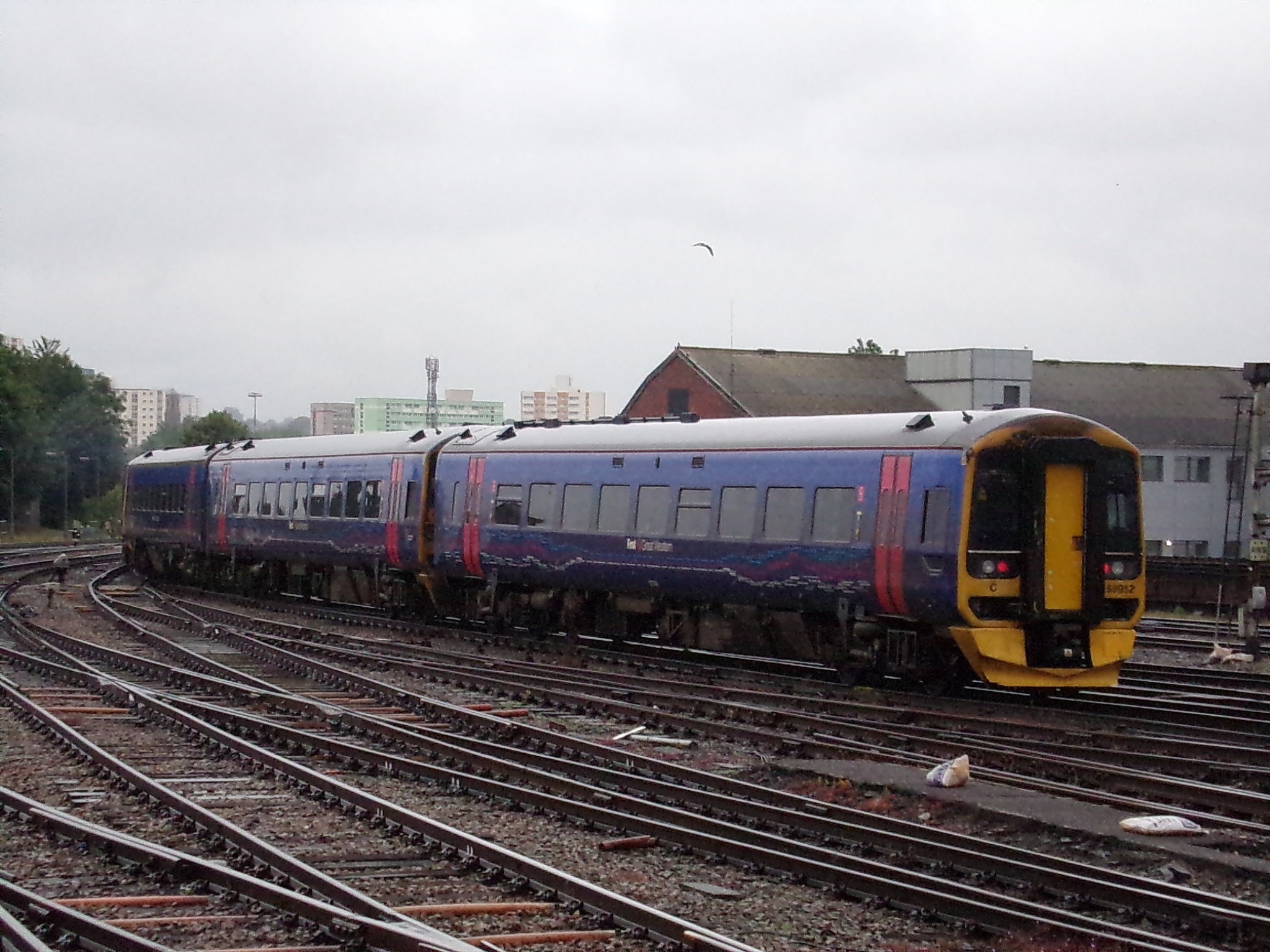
[76,455,102,527]
[0,446,16,539]
[244,392,261,441]
[45,449,69,543]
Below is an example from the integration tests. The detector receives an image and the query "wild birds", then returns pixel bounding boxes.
[693,243,714,257]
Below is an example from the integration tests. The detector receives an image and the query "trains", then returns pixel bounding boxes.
[118,404,1152,700]
